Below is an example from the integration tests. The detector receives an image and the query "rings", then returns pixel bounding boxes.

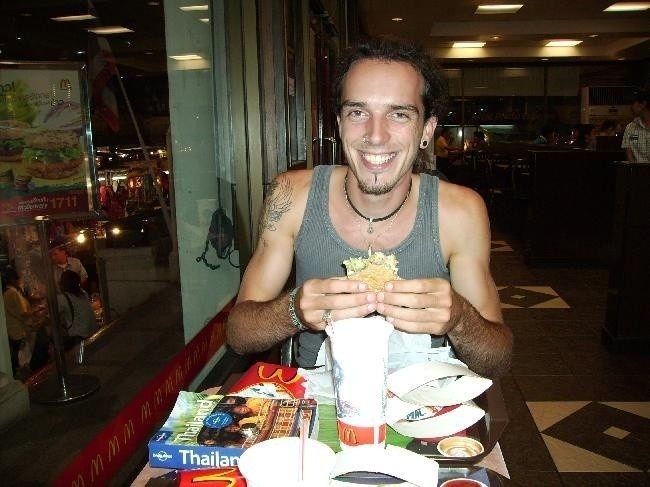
[322,311,333,325]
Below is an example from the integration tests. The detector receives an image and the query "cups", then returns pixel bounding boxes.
[237,436,335,487]
[324,315,394,454]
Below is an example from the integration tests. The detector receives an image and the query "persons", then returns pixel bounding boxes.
[224,42,513,377]
[141,186,145,203]
[2,265,44,377]
[135,187,140,203]
[527,128,547,144]
[51,270,97,352]
[47,245,87,295]
[436,130,461,175]
[621,94,650,161]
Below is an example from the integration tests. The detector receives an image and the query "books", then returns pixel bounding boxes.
[150,392,316,470]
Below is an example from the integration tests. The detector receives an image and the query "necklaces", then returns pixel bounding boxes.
[352,220,400,252]
[343,190,410,234]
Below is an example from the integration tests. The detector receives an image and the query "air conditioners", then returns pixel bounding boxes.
[580,86,637,124]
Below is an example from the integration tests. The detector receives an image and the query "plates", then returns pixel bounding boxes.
[303,376,509,468]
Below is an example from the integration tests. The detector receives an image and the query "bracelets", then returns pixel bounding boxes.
[287,289,303,330]
